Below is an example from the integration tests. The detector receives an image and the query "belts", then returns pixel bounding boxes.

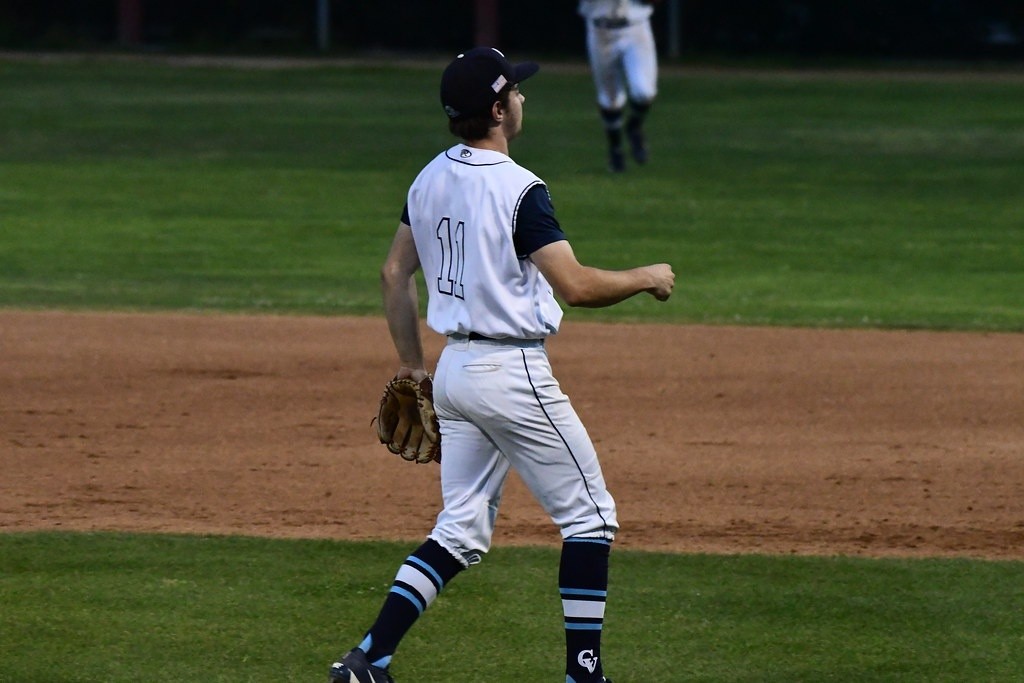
[593,17,628,30]
[468,331,545,343]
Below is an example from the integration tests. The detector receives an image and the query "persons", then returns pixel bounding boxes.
[578,0,659,174]
[327,44,677,683]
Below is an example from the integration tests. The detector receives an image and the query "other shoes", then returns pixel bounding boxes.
[606,127,624,169]
[625,125,646,164]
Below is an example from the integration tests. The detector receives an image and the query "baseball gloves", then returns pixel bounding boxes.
[367,371,444,466]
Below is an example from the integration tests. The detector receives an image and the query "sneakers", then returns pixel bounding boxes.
[329,647,394,683]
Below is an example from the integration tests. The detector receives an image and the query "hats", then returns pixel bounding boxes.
[439,47,540,126]
